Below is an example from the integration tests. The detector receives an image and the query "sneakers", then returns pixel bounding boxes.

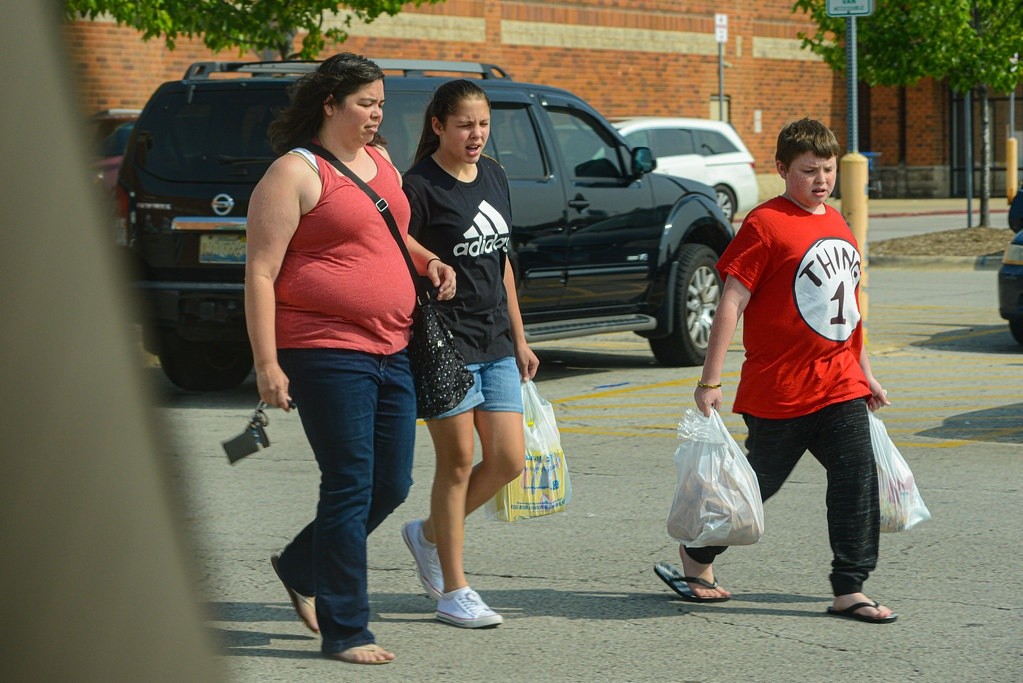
[402,519,444,599]
[436,586,504,628]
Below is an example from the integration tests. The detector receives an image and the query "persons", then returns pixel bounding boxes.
[652,118,900,623]
[223,54,457,663]
[402,79,540,629]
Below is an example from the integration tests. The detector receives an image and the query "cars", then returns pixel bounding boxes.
[87,106,147,225]
[995,186,1023,349]
[528,113,759,231]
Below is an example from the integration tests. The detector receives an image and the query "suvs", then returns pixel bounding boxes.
[110,58,736,393]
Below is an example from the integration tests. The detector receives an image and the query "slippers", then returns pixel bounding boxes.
[828,600,898,623]
[328,643,395,664]
[654,562,730,602]
[271,554,321,635]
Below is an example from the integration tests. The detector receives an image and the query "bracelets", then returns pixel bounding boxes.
[697,381,722,389]
[427,258,441,269]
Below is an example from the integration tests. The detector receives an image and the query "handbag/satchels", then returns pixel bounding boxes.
[485,380,571,523]
[666,404,764,548]
[865,390,931,532]
[417,291,475,418]
